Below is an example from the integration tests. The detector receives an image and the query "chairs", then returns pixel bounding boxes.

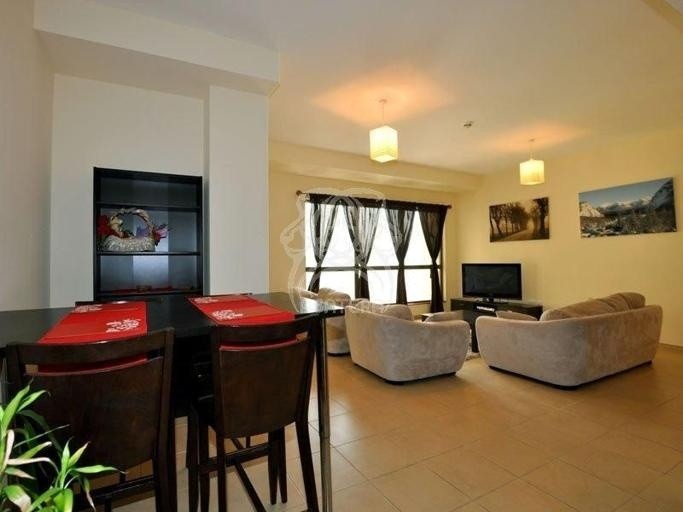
[7,325,180,512]
[187,309,323,512]
[74,296,164,307]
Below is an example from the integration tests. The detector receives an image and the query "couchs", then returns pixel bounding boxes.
[344,298,470,383]
[289,285,370,355]
[476,292,663,388]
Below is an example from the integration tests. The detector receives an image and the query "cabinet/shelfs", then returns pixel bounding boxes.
[92,166,203,300]
[450,297,543,321]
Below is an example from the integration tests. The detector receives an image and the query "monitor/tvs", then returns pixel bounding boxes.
[462,264,521,304]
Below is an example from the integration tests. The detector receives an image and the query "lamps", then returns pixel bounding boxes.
[520,159,545,185]
[369,126,398,164]
